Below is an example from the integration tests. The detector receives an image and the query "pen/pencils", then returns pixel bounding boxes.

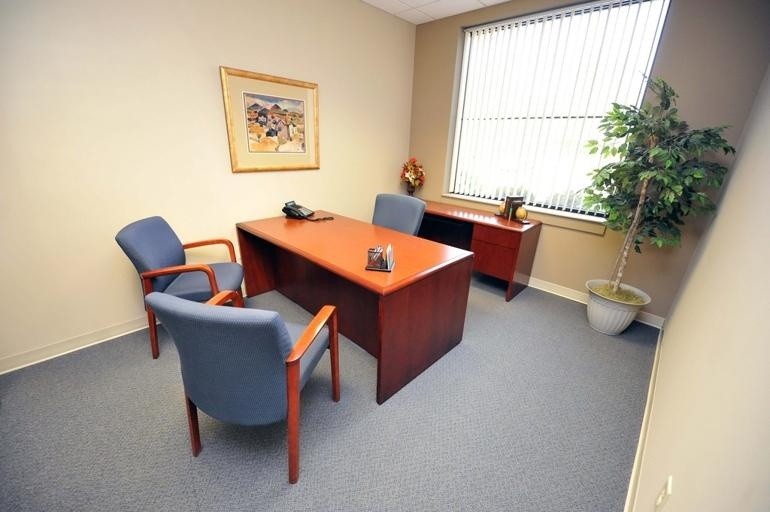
[371,245,383,262]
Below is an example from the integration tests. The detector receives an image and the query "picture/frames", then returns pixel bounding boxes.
[219,65,321,173]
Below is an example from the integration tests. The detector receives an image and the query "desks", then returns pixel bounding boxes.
[406,189,544,303]
[236,210,476,406]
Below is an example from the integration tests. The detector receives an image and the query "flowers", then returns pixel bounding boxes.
[400,159,426,190]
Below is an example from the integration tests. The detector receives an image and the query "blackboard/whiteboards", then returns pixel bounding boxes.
[282,201,314,219]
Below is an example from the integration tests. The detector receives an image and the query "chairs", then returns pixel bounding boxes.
[114,215,246,358]
[372,194,426,238]
[142,290,343,483]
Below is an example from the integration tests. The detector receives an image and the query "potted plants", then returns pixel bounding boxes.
[581,70,736,337]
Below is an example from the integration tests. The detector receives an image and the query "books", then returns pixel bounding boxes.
[504,196,523,219]
[509,200,525,221]
